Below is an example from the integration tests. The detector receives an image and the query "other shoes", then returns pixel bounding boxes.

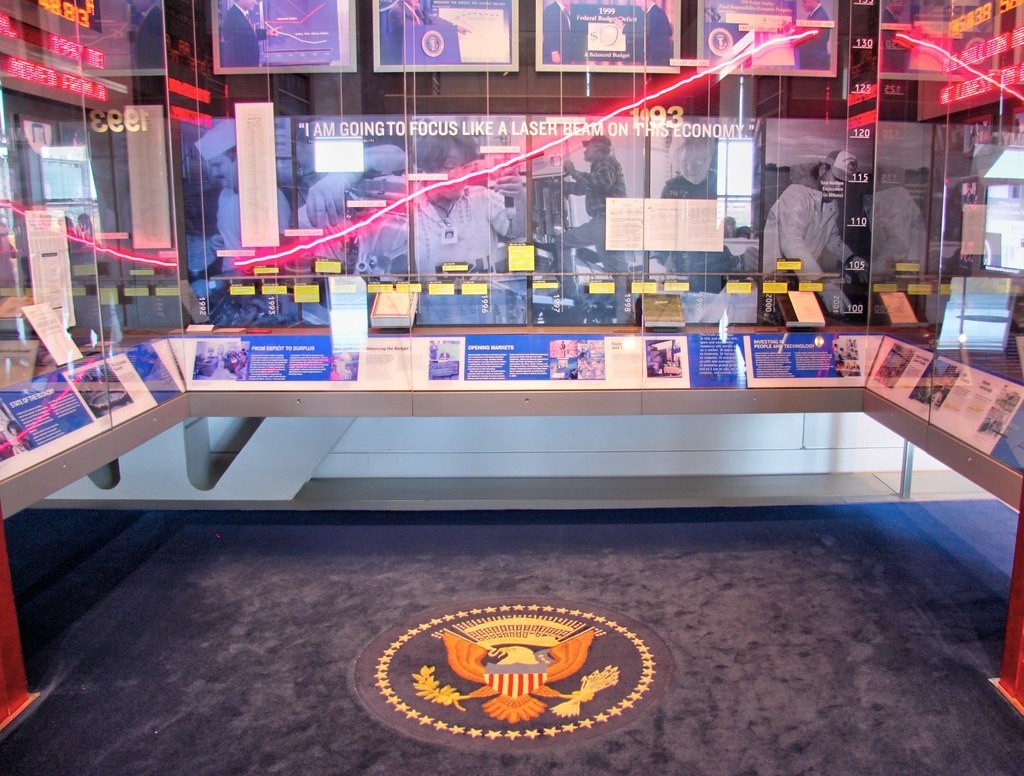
[549,282,576,299]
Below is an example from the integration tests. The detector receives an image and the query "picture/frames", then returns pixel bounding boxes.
[535,0,682,75]
[696,1,838,78]
[211,0,359,74]
[372,0,519,73]
[879,0,1000,83]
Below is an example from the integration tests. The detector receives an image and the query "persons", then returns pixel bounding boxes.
[194,114,406,276]
[967,120,989,151]
[331,364,357,381]
[861,157,927,276]
[388,0,429,33]
[881,0,925,72]
[57,213,93,248]
[578,349,593,371]
[218,0,281,67]
[401,129,526,277]
[430,341,450,360]
[570,369,577,379]
[543,0,574,64]
[833,338,861,377]
[614,0,672,66]
[229,349,247,379]
[763,150,869,313]
[785,0,830,65]
[648,138,761,293]
[932,387,944,404]
[557,135,628,324]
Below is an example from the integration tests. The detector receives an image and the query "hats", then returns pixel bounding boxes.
[825,150,858,182]
[582,135,611,148]
[193,120,236,161]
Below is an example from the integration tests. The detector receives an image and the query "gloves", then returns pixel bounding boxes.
[851,256,869,284]
[817,282,852,315]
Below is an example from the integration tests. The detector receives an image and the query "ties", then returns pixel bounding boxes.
[246,14,257,38]
